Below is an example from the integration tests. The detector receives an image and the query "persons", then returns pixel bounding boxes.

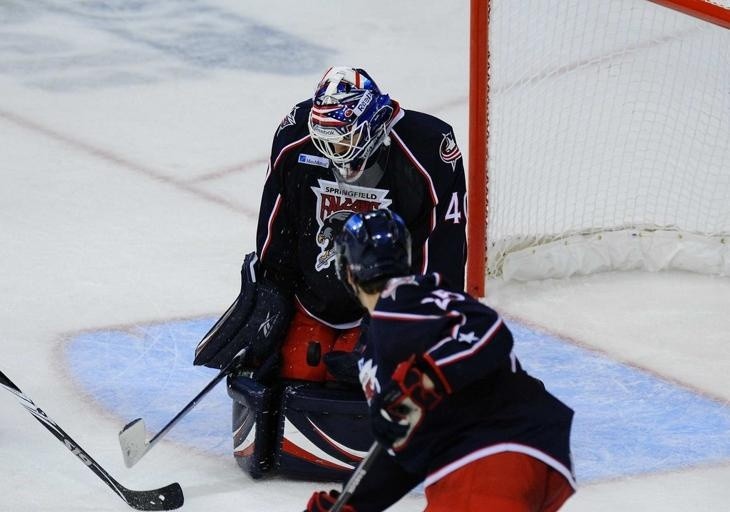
[193,66,467,482]
[305,208,576,512]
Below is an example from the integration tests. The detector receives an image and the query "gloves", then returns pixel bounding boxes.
[304,489,375,512]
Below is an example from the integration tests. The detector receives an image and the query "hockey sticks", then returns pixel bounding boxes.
[0,370,184,511]
[119,342,251,469]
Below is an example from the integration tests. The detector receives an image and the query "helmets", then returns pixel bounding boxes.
[308,66,393,184]
[335,208,412,292]
